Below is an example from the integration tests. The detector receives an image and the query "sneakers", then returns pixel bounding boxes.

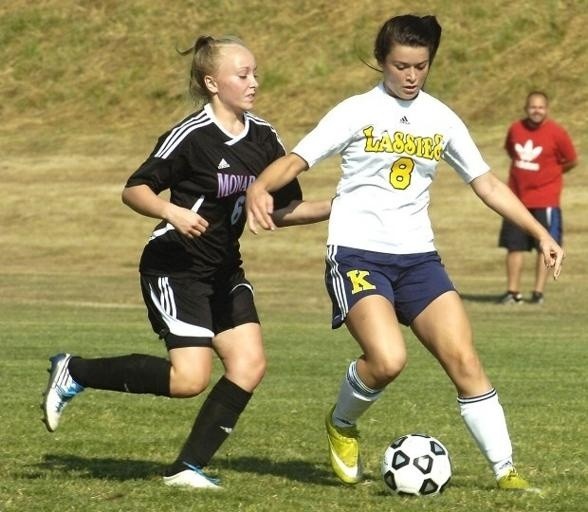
[496,469,546,498]
[496,291,544,304]
[325,403,363,485]
[40,353,84,433]
[162,463,225,490]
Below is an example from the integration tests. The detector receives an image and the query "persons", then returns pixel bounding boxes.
[244,13,564,491]
[38,35,335,489]
[498,92,580,305]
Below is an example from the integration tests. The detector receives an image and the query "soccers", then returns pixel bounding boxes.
[382,434,452,499]
